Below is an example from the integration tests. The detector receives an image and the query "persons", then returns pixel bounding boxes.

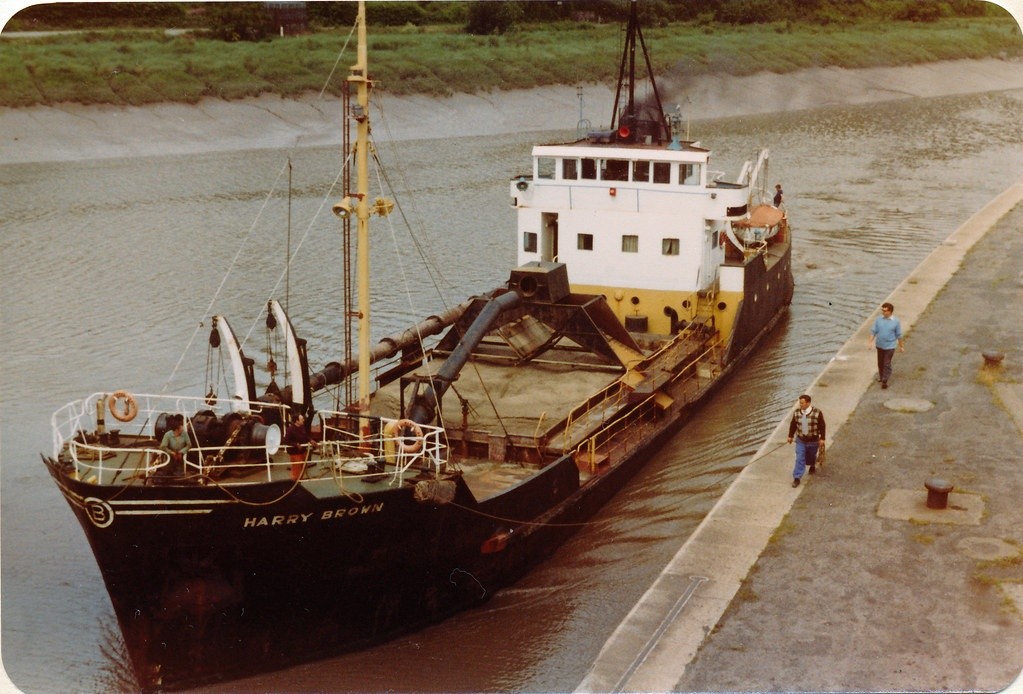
[868,303,905,389]
[774,185,785,209]
[788,395,826,488]
[284,413,320,482]
[159,420,192,474]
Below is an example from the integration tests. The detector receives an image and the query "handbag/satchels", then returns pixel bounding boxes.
[815,440,826,462]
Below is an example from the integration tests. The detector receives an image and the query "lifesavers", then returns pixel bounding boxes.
[108,391,138,422]
[391,418,424,453]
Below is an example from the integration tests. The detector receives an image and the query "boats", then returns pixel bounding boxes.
[34,3,796,693]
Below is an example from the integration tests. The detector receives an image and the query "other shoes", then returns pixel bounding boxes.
[791,478,800,488]
[881,382,887,389]
[809,465,815,475]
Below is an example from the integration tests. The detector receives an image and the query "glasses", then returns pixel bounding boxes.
[881,309,890,312]
